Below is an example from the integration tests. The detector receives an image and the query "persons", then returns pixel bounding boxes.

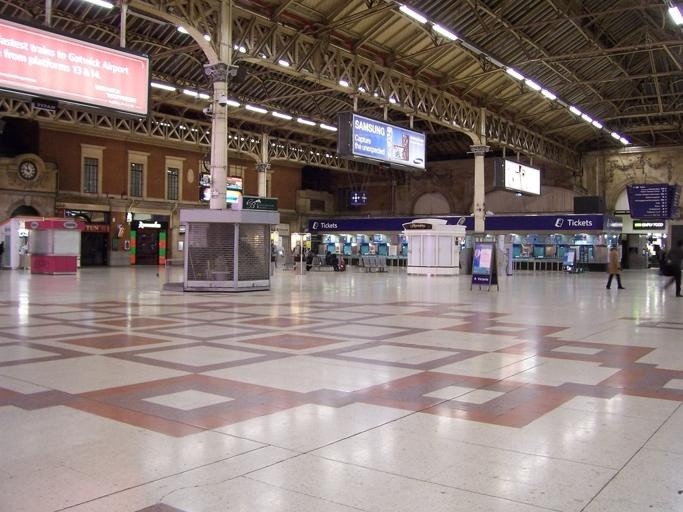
[658,239,683,298]
[603,243,624,290]
[304,247,345,272]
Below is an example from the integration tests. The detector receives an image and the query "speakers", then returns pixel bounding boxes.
[573,196,606,214]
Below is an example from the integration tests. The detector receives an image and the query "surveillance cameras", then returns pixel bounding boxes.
[202,108,210,113]
[217,98,227,107]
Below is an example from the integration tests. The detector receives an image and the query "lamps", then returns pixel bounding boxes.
[150,80,174,92]
[397,0,631,146]
[664,1,683,32]
[85,1,115,11]
[178,85,337,136]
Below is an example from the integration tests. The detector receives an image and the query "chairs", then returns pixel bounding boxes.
[305,255,334,272]
[357,256,388,273]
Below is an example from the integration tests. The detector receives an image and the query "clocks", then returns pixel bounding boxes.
[20,161,37,180]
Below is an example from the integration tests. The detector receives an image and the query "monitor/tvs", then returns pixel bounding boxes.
[512,246,592,257]
[199,172,244,204]
[328,245,408,254]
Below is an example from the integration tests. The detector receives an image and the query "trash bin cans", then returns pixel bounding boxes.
[331,254,344,271]
